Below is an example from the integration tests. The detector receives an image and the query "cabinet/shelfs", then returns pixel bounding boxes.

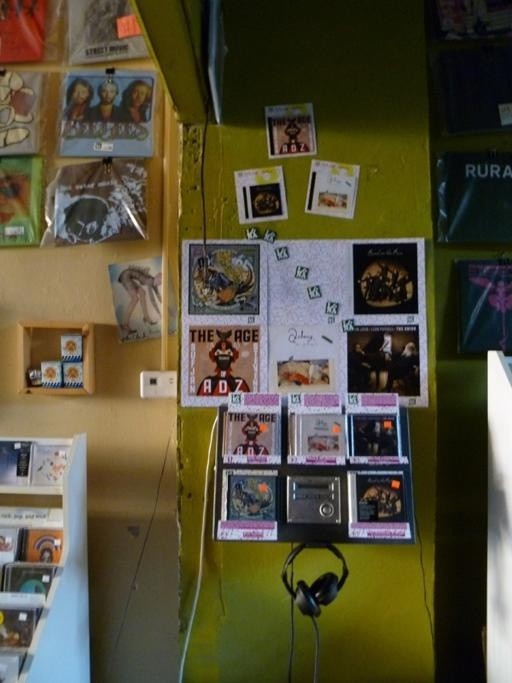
[14,318,97,398]
[485,348,512,682]
[0,432,93,681]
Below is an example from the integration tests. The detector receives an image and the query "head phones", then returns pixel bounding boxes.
[282,544,349,617]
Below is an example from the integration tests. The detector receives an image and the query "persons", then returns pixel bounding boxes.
[63,78,151,124]
[356,259,412,304]
[118,263,162,334]
[348,325,420,394]
